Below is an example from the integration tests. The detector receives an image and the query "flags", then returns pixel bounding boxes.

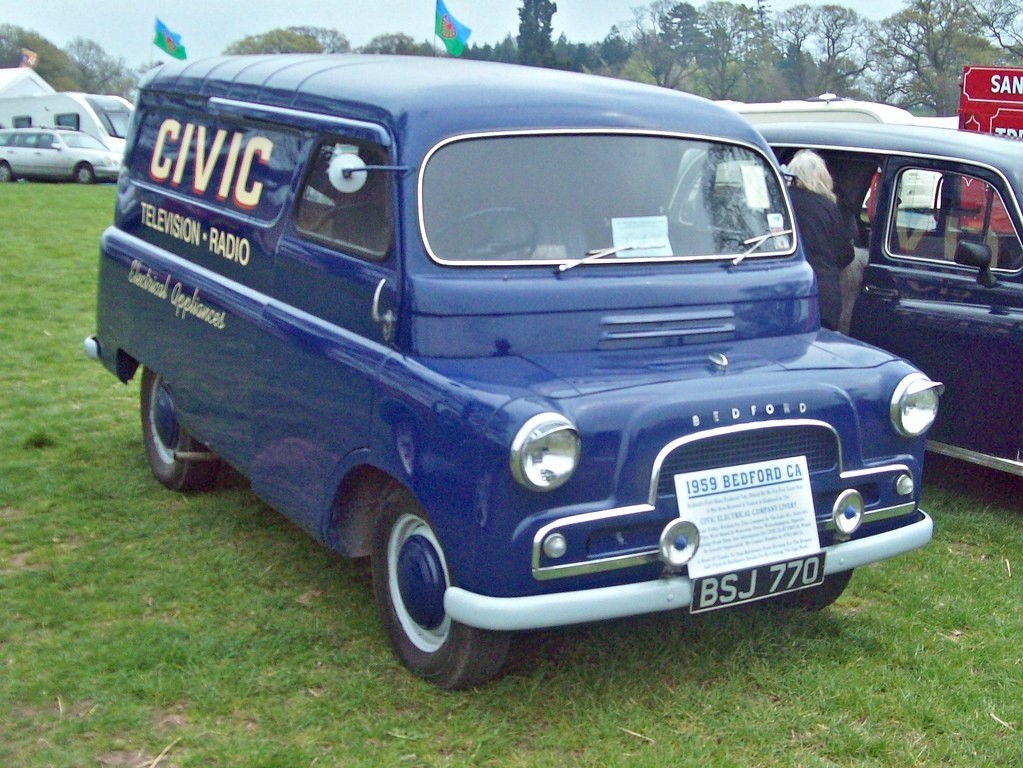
[19,47,37,68]
[152,19,186,60]
[434,0,471,58]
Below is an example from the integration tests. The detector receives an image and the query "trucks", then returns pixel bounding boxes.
[0,92,136,155]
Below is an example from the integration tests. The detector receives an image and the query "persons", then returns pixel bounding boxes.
[783,147,857,330]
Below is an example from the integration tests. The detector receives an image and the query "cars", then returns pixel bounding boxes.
[664,91,1023,475]
[81,54,945,692]
[0,128,123,185]
